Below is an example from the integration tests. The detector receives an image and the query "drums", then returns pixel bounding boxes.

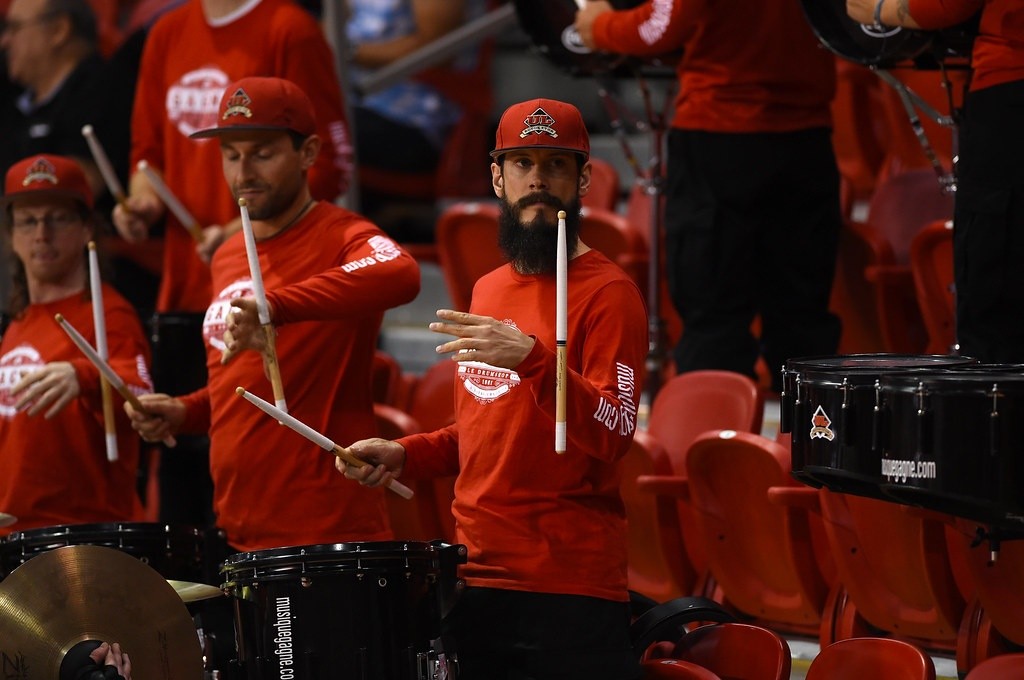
[0,519,233,680]
[797,0,985,68]
[507,0,686,81]
[887,363,1024,537]
[220,538,472,680]
[780,351,983,507]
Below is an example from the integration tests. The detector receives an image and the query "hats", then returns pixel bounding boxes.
[186,77,320,138]
[3,153,96,210]
[490,99,590,162]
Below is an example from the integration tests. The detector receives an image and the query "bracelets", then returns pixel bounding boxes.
[873,1,886,32]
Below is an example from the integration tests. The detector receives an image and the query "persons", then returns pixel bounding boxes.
[336,99,648,679]
[121,75,421,680]
[0,155,160,527]
[68,641,132,680]
[0,0,1023,464]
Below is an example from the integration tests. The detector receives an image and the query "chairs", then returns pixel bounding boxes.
[94,0,1024,680]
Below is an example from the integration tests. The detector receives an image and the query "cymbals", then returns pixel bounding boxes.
[0,543,205,680]
